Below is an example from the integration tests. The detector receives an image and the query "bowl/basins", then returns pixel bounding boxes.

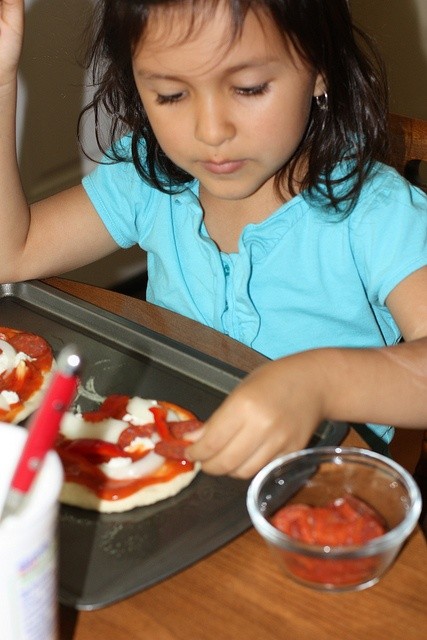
[246,444,424,596]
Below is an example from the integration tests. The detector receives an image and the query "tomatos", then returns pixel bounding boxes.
[271,493,387,546]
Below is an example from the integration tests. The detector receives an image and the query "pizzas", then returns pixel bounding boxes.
[55,393,205,514]
[0,326,57,425]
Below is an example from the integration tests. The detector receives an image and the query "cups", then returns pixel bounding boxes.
[0,423,64,639]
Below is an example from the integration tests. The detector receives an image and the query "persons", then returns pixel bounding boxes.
[0,1,427,482]
